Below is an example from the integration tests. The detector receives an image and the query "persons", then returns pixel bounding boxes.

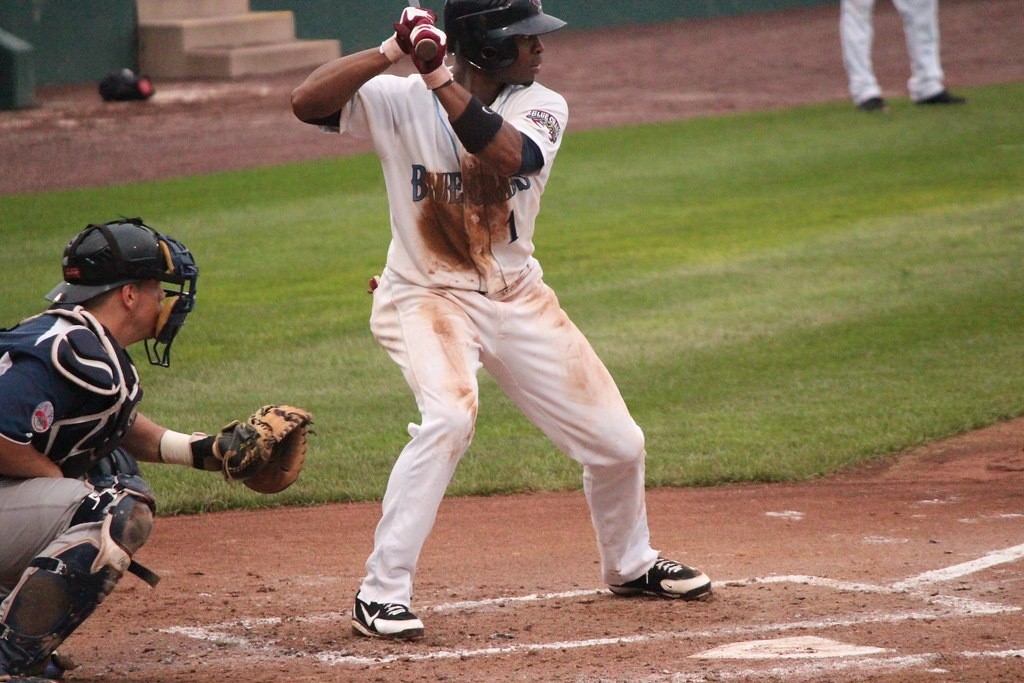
[2,216,308,683]
[836,0,969,115]
[288,0,715,640]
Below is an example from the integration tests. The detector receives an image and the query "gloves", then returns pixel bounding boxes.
[392,22,454,90]
[379,6,438,64]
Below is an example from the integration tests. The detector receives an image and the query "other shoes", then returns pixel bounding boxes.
[918,91,966,105]
[859,97,895,115]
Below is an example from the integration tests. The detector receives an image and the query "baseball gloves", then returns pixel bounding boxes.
[210,403,318,494]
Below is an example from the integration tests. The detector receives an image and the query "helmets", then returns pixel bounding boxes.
[44,218,185,304]
[444,0,566,54]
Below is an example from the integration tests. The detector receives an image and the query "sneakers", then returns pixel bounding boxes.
[351,588,426,642]
[607,555,712,601]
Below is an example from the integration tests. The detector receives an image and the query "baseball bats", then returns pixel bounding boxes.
[408,1,438,61]
